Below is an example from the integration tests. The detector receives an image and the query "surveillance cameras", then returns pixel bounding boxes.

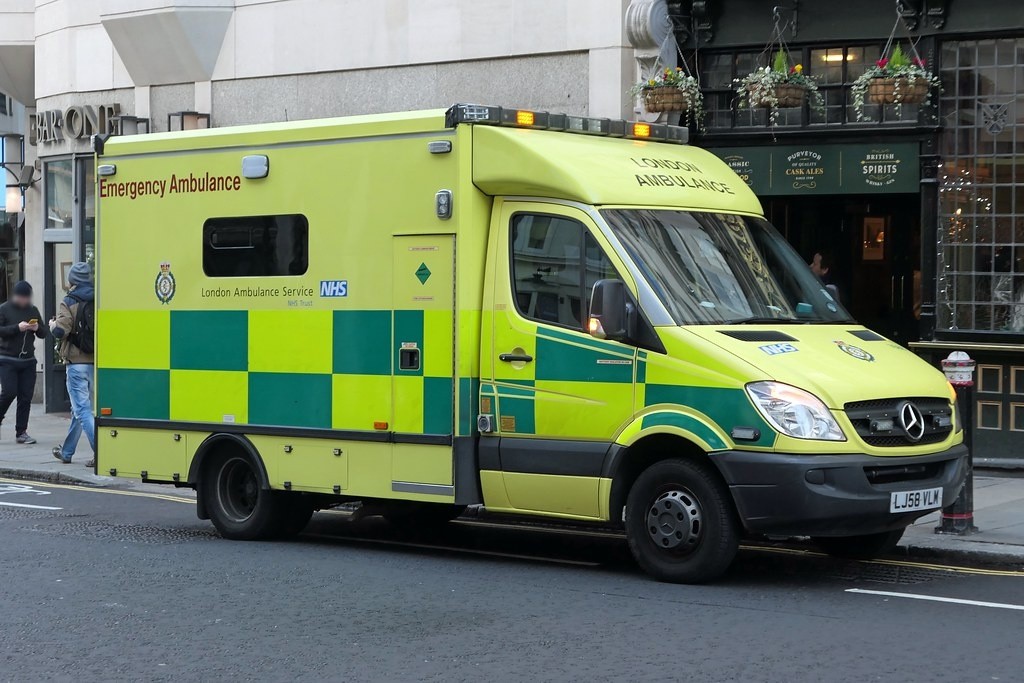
[20,166,34,188]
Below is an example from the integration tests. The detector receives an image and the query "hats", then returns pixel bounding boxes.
[68,262,91,286]
[14,281,32,297]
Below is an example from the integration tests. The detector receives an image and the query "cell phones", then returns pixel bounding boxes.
[29,319,39,325]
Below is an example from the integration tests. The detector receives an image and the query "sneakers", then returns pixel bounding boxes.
[85,458,95,467]
[52,444,71,463]
[17,432,36,444]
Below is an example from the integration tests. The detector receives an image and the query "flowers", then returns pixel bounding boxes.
[727,50,827,144]
[851,41,945,123]
[625,66,708,136]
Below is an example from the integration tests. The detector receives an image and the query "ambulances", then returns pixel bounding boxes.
[94,104,972,584]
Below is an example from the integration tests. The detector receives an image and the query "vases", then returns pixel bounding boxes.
[748,84,808,108]
[867,76,930,104]
[642,86,695,111]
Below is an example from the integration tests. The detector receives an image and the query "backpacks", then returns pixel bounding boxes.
[64,294,94,354]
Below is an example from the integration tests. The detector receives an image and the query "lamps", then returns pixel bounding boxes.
[941,351,977,386]
[4,136,22,213]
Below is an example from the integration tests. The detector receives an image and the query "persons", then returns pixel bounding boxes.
[48,263,95,467]
[807,251,848,321]
[0,280,48,444]
